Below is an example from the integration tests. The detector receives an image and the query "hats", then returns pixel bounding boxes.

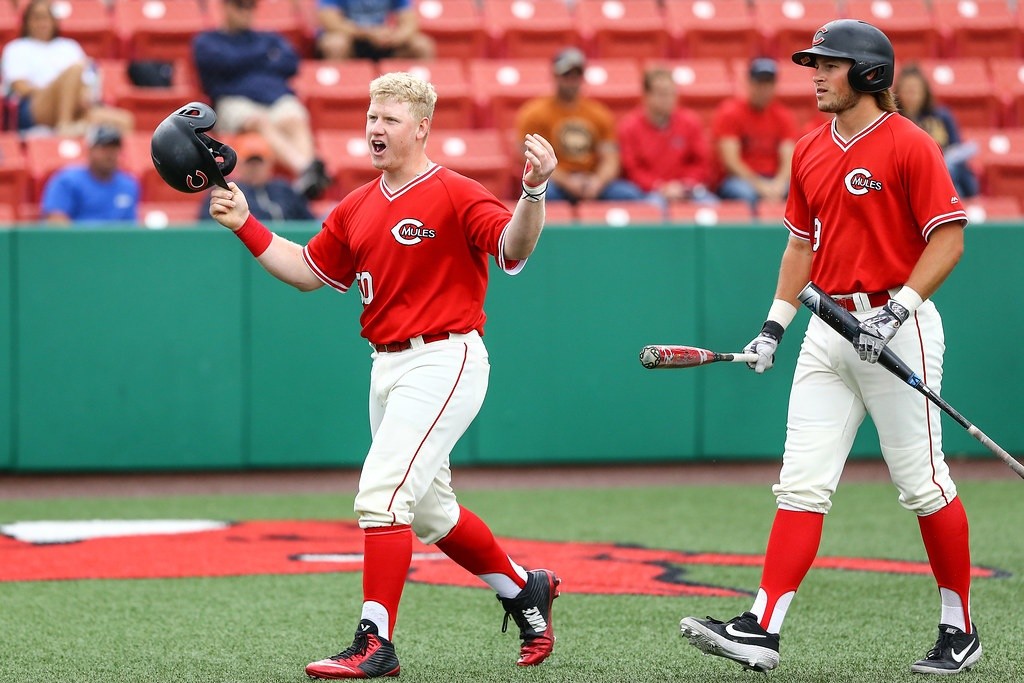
[553,47,585,75]
[748,56,778,80]
[88,123,122,149]
[233,133,272,157]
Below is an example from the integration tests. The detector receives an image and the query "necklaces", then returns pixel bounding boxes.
[415,159,431,177]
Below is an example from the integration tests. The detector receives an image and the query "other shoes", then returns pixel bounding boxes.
[297,155,327,201]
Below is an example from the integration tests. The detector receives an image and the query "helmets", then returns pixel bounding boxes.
[150,102,237,194]
[792,19,894,93]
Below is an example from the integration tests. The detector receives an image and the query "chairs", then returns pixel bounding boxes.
[1,0,1024,229]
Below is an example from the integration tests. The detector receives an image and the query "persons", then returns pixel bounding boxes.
[677,19,985,675]
[314,0,437,61]
[190,0,332,221]
[0,0,102,134]
[40,122,141,227]
[208,73,562,679]
[513,50,979,202]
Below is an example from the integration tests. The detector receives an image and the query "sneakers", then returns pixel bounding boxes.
[679,611,780,673]
[911,622,982,674]
[495,569,561,667]
[306,619,400,680]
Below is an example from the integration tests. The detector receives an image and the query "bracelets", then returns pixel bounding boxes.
[520,179,549,201]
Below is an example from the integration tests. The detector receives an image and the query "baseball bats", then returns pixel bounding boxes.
[638,344,775,370]
[795,280,1024,481]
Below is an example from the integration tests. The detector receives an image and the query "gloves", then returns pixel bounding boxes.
[742,320,785,374]
[853,299,910,364]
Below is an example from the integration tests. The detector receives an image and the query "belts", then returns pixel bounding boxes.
[376,333,449,353]
[832,291,892,313]
